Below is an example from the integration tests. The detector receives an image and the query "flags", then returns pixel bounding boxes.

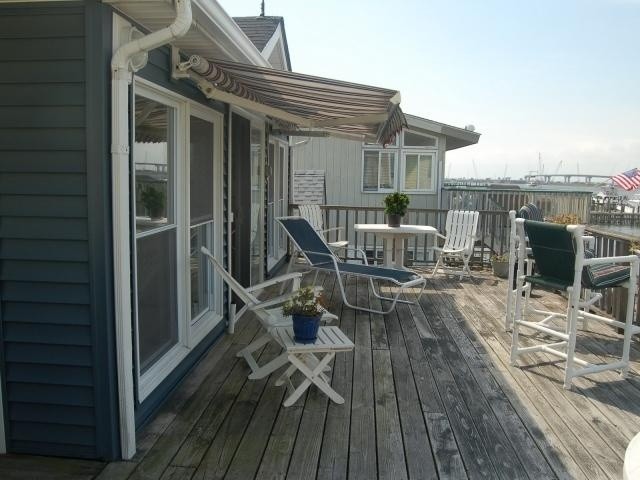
[611,167,640,192]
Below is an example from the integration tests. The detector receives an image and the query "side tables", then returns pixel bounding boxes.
[272,325,351,406]
[353,222,437,268]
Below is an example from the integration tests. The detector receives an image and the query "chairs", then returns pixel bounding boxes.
[429,210,480,282]
[202,248,339,382]
[276,205,426,314]
[502,203,637,396]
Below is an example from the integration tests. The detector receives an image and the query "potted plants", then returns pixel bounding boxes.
[280,284,326,342]
[382,191,410,226]
[491,253,514,277]
